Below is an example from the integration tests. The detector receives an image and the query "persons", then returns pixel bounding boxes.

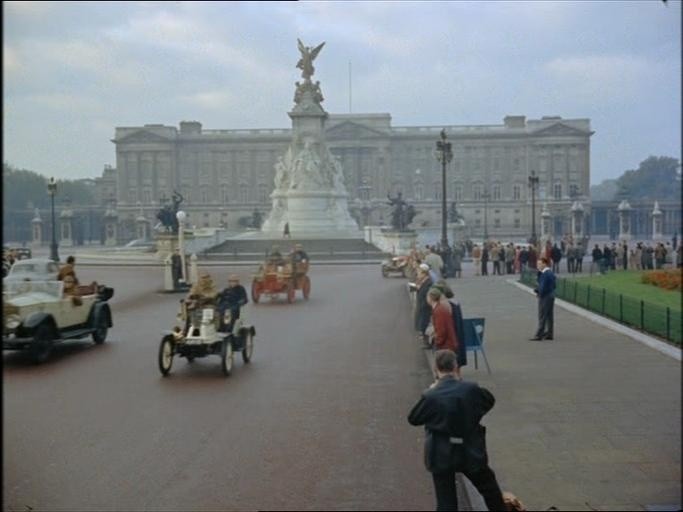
[180,272,248,332]
[264,243,309,286]
[57,256,99,295]
[407,349,505,510]
[466,235,682,275]
[164,189,184,228]
[408,241,467,388]
[385,190,464,232]
[310,81,321,101]
[528,257,556,341]
[292,81,303,104]
[300,47,314,76]
[170,247,183,289]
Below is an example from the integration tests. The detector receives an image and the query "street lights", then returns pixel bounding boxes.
[480,188,491,241]
[46,176,62,260]
[526,168,538,247]
[434,128,453,259]
[175,210,190,282]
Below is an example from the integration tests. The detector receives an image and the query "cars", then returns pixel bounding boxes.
[2,259,60,288]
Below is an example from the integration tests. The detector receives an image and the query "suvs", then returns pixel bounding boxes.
[0,258,113,364]
